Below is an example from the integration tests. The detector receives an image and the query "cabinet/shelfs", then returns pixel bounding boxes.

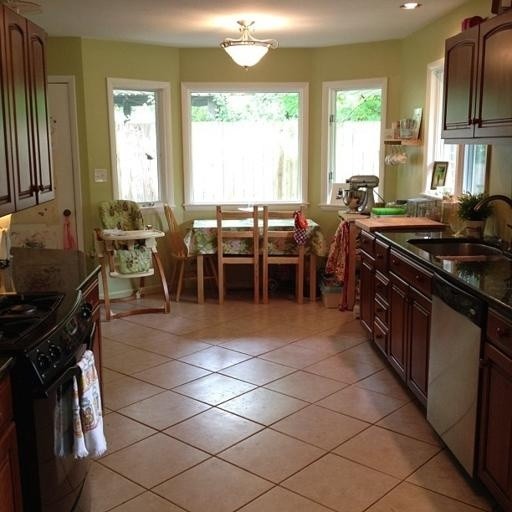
[0,245,101,508]
[2,5,55,215]
[440,11,511,145]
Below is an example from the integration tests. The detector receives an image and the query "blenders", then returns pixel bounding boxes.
[342,175,379,215]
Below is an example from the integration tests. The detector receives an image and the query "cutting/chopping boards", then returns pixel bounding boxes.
[355,217,446,233]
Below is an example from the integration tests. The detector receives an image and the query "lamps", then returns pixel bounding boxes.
[220,21,279,69]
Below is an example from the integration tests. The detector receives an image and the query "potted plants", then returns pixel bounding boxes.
[456,191,492,235]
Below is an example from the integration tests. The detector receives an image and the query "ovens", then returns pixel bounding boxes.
[23,291,96,511]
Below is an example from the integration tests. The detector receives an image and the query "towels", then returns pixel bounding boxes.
[64,215,76,250]
[53,348,107,461]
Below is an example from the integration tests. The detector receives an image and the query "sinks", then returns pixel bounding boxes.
[434,256,511,262]
[405,236,503,256]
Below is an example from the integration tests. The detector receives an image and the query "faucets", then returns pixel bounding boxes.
[474,193,512,212]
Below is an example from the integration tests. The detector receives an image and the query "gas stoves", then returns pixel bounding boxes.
[0,291,65,346]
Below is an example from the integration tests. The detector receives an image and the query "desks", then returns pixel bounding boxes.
[192,218,320,302]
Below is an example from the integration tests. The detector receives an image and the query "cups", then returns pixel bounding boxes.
[385,151,410,167]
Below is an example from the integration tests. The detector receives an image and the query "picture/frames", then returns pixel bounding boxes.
[430,161,448,191]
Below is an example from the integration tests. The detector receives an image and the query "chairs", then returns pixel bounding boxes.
[93,199,171,319]
[163,203,225,305]
[216,204,259,303]
[263,205,305,303]
